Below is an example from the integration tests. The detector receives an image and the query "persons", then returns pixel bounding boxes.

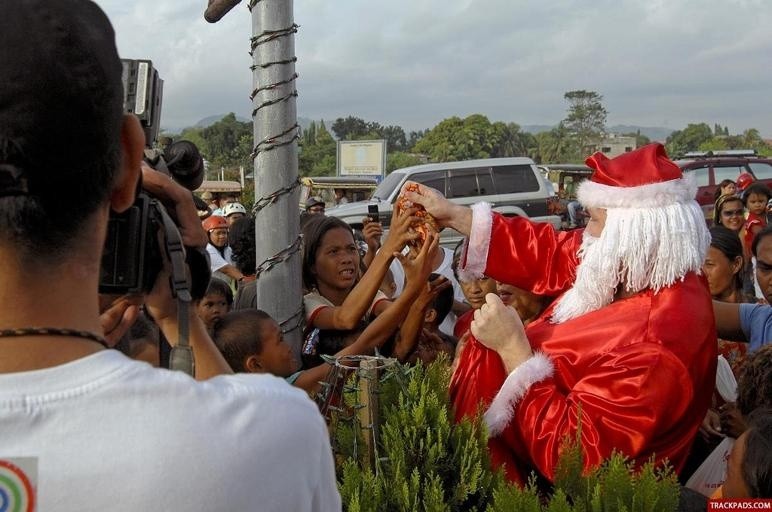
[1,0,343,511]
[702,173,769,512]
[334,188,348,205]
[305,197,325,216]
[315,276,452,393]
[200,277,233,336]
[452,280,540,375]
[207,232,440,393]
[198,192,256,311]
[451,238,497,338]
[302,202,440,371]
[362,216,473,368]
[399,144,718,498]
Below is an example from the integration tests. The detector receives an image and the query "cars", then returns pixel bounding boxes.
[669,148,772,223]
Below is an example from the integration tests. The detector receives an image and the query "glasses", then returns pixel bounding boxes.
[309,207,325,212]
[723,209,743,216]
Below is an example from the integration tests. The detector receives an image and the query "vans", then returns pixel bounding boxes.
[322,155,563,256]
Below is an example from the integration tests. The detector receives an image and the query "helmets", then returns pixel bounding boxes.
[200,215,227,232]
[220,202,246,218]
[304,196,325,212]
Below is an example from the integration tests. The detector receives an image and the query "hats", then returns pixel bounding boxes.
[0,1,124,198]
[575,141,698,212]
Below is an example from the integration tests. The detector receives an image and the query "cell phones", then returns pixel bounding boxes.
[367,203,380,223]
